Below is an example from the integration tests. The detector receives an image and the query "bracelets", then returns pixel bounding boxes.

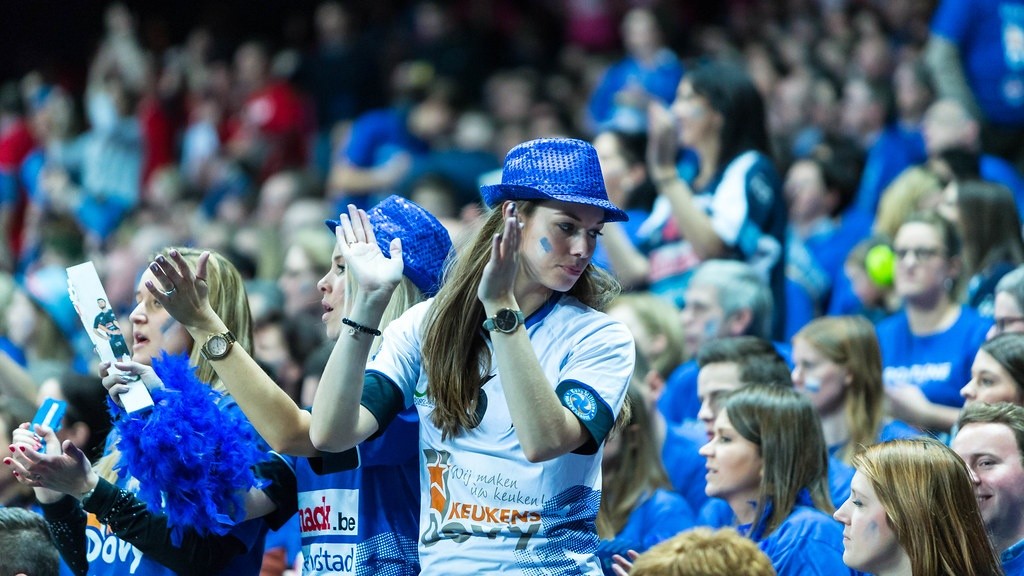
[342,318,381,336]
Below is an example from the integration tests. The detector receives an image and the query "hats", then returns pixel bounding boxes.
[325,195,458,297]
[994,266,1024,311]
[481,138,629,222]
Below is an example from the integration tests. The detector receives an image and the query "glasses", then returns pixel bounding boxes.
[890,248,942,260]
[992,317,1024,331]
[944,201,955,208]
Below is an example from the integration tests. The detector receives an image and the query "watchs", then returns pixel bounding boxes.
[78,489,94,508]
[482,307,525,334]
[199,330,237,360]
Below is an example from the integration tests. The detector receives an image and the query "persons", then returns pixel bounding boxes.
[628,527,778,576]
[93,298,130,362]
[832,436,1006,576]
[99,195,459,576]
[1,245,267,576]
[611,383,875,576]
[790,314,926,508]
[949,401,1024,576]
[309,137,637,576]
[693,336,794,530]
[959,330,1024,409]
[594,383,694,549]
[0,0,1023,576]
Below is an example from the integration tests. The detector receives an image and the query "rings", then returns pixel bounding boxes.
[166,288,175,295]
[348,241,354,246]
[36,475,41,480]
